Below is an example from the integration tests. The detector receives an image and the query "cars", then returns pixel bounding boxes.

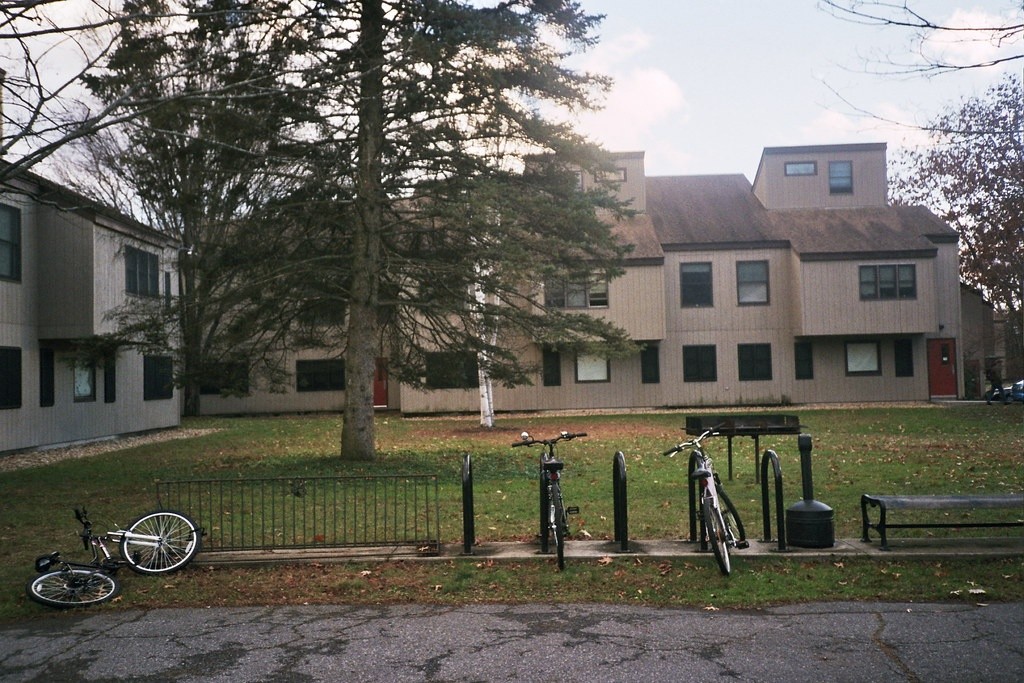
[991,379,1024,404]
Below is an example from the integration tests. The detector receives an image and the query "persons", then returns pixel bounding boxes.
[986,360,1011,405]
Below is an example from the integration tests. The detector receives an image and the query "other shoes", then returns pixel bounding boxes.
[1004,402,1012,405]
[986,401,993,406]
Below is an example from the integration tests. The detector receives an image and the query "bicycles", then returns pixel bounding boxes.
[511,430,588,571]
[25,503,202,610]
[662,421,750,576]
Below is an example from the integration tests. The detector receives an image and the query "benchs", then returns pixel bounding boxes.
[862,492,1024,551]
